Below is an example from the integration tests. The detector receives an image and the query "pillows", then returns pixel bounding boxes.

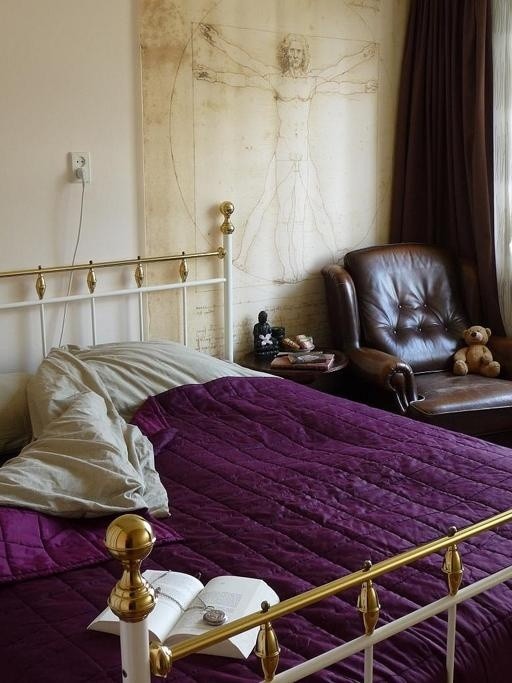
[1,367,37,447]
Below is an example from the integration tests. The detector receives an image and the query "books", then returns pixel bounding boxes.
[271,353,334,371]
[86,569,281,662]
[288,351,326,364]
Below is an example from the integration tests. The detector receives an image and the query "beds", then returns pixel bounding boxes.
[0,200,511,683]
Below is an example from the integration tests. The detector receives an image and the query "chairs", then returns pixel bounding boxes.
[318,236,511,449]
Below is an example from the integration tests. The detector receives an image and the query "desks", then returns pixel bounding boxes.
[239,345,348,391]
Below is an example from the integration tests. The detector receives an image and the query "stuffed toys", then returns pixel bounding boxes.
[451,326,501,378]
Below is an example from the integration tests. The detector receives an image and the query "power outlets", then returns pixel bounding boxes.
[67,150,92,184]
